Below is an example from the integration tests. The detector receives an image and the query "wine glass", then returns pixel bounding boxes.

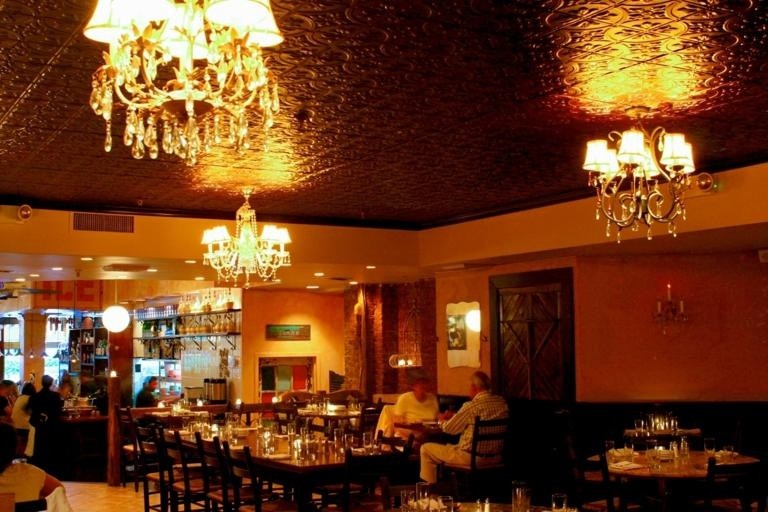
[604,412,741,471]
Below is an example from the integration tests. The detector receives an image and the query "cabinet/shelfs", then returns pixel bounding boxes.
[179,309,242,349]
[142,316,181,359]
[68,327,132,395]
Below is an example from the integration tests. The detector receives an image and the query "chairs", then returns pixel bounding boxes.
[113,389,768,512]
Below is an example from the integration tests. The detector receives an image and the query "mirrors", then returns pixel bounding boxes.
[445,301,482,369]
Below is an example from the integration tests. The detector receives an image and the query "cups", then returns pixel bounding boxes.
[398,476,569,512]
[171,392,381,465]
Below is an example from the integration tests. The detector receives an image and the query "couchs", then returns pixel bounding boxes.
[40,414,168,481]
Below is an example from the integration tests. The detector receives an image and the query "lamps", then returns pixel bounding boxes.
[649,280,688,335]
[101,279,131,333]
[17,204,33,221]
[200,187,293,289]
[0,304,61,358]
[695,172,715,192]
[583,122,696,243]
[82,0,285,166]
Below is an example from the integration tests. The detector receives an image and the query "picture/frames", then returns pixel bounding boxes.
[448,314,467,351]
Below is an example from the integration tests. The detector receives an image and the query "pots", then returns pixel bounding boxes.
[203,377,228,405]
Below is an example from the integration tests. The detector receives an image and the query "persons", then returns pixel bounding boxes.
[0,380,16,418]
[420,371,509,486]
[8,385,19,401]
[11,383,36,464]
[393,375,440,453]
[24,375,62,467]
[136,376,162,428]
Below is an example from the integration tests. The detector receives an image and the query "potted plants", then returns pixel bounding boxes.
[96,339,108,356]
